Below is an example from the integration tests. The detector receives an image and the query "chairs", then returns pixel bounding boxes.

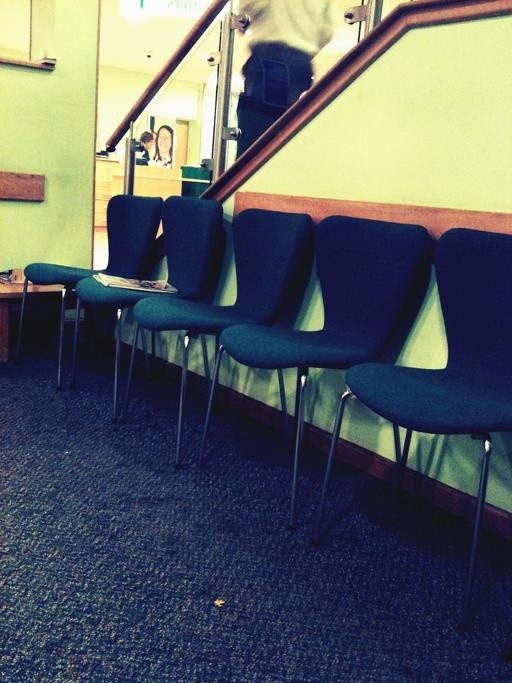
[310,229,510,636]
[119,208,313,472]
[17,195,165,394]
[69,198,225,422]
[192,214,432,536]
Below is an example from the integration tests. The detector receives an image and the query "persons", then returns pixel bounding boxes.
[140,128,157,161]
[154,125,173,168]
[229,0,334,164]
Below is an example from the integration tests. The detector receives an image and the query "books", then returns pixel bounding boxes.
[93,271,178,295]
